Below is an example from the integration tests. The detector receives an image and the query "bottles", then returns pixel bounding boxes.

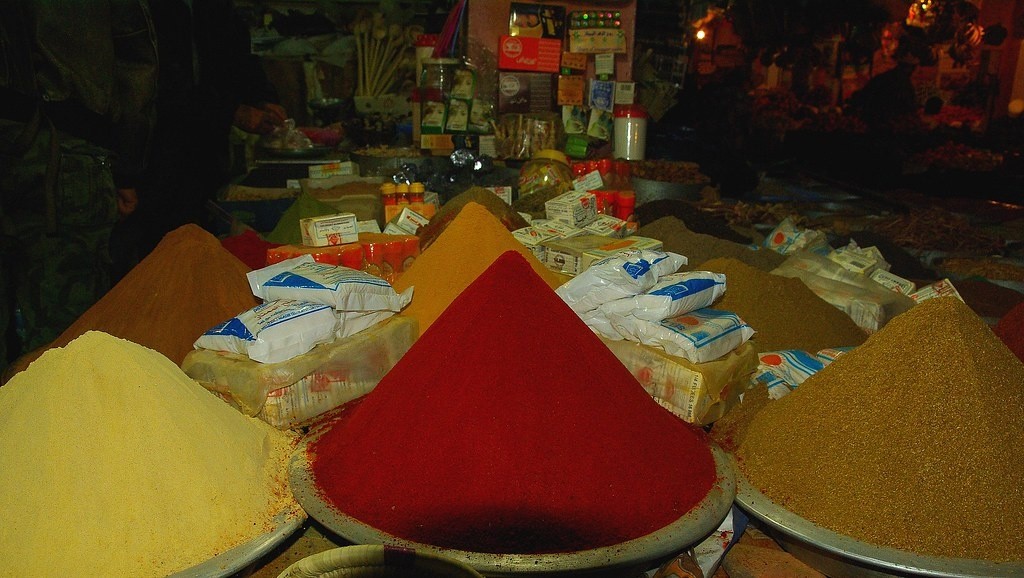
[381,182,425,224]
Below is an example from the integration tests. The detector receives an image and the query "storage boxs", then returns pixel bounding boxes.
[177,136,760,430]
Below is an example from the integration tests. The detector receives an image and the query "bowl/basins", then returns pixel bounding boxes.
[633,169,712,202]
[164,498,307,578]
[728,452,1024,578]
[251,142,335,184]
[287,418,736,572]
[351,147,426,178]
